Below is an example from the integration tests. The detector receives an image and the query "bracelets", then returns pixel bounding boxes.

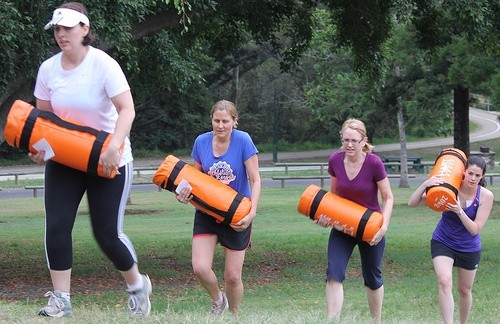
[457,209,464,216]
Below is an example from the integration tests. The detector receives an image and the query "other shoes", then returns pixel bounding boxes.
[210,294,228,315]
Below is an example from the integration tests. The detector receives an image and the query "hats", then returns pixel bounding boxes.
[43,8,89,31]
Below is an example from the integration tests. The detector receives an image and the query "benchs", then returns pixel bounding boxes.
[275,162,435,176]
[0,168,158,185]
[272,174,416,189]
[24,182,161,197]
[483,174,500,186]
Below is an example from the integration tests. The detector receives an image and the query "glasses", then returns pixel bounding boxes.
[340,138,363,145]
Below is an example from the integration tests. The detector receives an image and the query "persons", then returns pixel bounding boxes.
[176,100,261,319]
[28,2,152,319]
[408,156,494,324]
[314,118,393,324]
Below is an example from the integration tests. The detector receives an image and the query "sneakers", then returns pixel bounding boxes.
[38,291,72,318]
[126,274,152,317]
